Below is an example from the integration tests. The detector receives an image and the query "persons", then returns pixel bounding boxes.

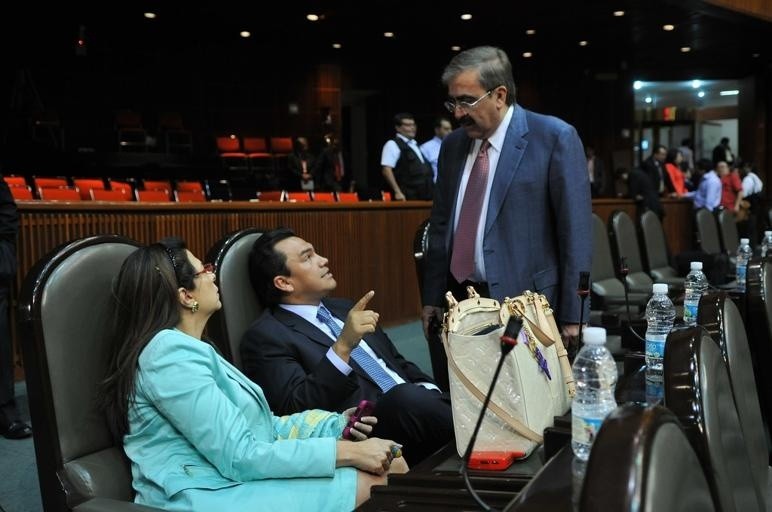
[421,45,591,356]
[380,112,435,202]
[611,128,767,243]
[292,106,346,190]
[420,119,454,183]
[100,236,413,511]
[237,228,462,462]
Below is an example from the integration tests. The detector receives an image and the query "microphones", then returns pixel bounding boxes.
[621,257,645,341]
[463,315,523,512]
[576,271,591,354]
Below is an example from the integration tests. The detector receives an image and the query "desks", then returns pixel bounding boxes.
[343,416,573,512]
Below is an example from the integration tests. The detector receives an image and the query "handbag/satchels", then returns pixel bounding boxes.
[441,286,576,461]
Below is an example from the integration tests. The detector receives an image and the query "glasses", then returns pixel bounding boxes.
[192,263,214,278]
[444,88,493,113]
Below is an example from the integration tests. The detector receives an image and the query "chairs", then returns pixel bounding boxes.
[134,187,172,202]
[108,178,133,195]
[587,211,642,327]
[607,207,678,309]
[693,203,744,284]
[284,191,312,203]
[636,206,686,299]
[38,186,81,202]
[269,135,297,176]
[33,177,69,199]
[73,178,105,200]
[662,322,762,511]
[14,231,169,511]
[2,176,27,188]
[142,180,172,195]
[242,135,273,175]
[310,191,336,203]
[745,253,771,427]
[7,183,33,201]
[204,224,269,376]
[215,136,249,178]
[173,189,207,203]
[89,188,132,202]
[578,400,717,512]
[176,180,203,192]
[255,190,285,203]
[335,192,360,203]
[694,291,772,511]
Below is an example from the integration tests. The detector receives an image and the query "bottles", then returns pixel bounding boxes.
[762,231,771,262]
[568,326,620,459]
[736,238,752,291]
[644,376,665,408]
[643,280,677,374]
[683,260,710,326]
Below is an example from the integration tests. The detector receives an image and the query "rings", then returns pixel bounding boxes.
[390,446,402,458]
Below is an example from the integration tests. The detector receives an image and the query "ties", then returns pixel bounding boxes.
[450,138,493,284]
[317,306,398,394]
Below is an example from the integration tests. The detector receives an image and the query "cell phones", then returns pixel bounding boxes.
[466,450,526,470]
[343,400,376,440]
